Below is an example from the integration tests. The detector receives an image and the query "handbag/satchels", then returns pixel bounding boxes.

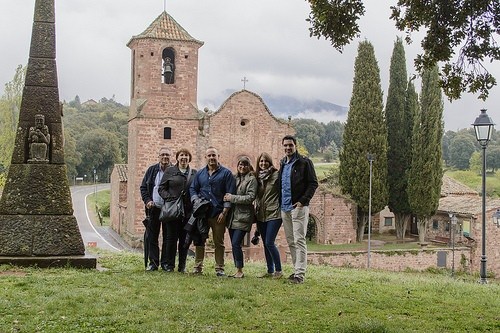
[159,197,185,223]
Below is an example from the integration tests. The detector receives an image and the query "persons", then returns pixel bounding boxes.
[223,156,258,278]
[140,148,179,272]
[158,149,198,274]
[279,136,318,284]
[29,114,50,160]
[190,146,236,276]
[163,57,174,84]
[255,152,283,280]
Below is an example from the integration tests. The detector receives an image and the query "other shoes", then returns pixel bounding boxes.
[273,272,283,279]
[164,264,174,272]
[289,273,304,284]
[216,270,225,276]
[257,274,274,278]
[146,264,158,271]
[193,268,201,274]
[228,272,244,278]
[178,268,188,275]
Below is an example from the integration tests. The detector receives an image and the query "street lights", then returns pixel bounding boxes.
[471,109,496,283]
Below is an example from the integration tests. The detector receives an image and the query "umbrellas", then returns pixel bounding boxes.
[251,229,261,245]
[142,209,153,272]
